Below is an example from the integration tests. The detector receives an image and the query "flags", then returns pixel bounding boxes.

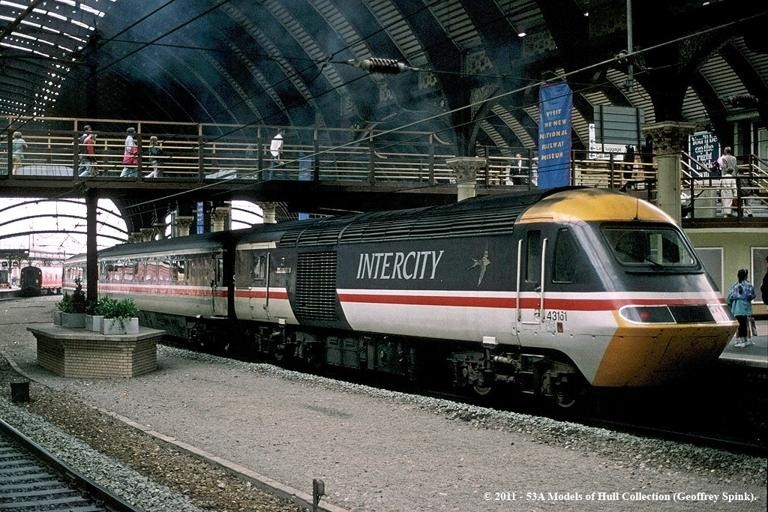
[535,81,572,190]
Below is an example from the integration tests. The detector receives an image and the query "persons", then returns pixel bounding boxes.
[84,132,98,175]
[735,165,754,216]
[759,256,768,305]
[119,127,138,177]
[679,184,697,219]
[719,167,737,218]
[712,145,738,218]
[144,136,163,178]
[725,269,756,347]
[9,131,28,175]
[269,127,290,181]
[78,125,97,176]
[509,153,530,184]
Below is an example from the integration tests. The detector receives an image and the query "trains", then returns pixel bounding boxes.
[63,182,745,422]
[15,264,64,295]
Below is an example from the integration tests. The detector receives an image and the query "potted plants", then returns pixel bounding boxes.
[53,275,140,335]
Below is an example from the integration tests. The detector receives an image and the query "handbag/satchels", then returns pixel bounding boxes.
[122,146,139,166]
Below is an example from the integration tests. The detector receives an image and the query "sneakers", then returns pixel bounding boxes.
[733,333,758,348]
